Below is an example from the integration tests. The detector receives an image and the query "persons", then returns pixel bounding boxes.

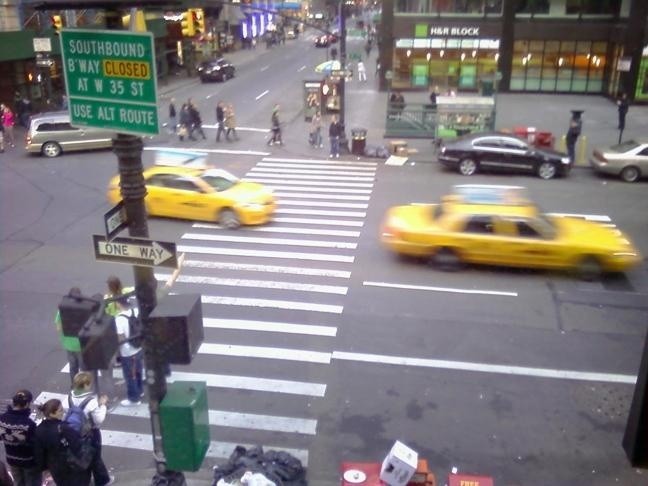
[330,84,341,106]
[430,85,441,105]
[268,110,284,146]
[357,59,368,81]
[0,88,69,154]
[154,252,185,385]
[308,91,321,110]
[390,93,397,120]
[52,274,146,417]
[64,372,116,486]
[167,96,242,143]
[36,397,97,486]
[0,389,42,484]
[396,89,405,120]
[565,118,582,168]
[327,114,342,158]
[309,111,324,149]
[616,92,628,129]
[244,27,286,49]
[266,102,284,145]
[444,87,456,98]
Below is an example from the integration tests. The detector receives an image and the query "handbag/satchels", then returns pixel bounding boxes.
[177,124,189,136]
[309,133,315,144]
[65,443,97,471]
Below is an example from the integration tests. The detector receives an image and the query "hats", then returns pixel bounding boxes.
[116,294,130,306]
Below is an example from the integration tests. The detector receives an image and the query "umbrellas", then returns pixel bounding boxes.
[315,60,341,74]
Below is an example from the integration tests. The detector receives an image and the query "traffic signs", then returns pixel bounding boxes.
[35,59,54,67]
[57,27,160,138]
[92,233,180,268]
[330,68,353,77]
[103,194,129,243]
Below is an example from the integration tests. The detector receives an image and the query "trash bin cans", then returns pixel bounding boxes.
[352,128,367,154]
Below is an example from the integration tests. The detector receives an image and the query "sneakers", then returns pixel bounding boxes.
[120,400,141,406]
[104,477,114,486]
[180,137,283,146]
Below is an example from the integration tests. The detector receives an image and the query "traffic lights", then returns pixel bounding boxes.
[192,9,204,35]
[179,9,194,36]
[52,14,62,35]
[76,316,116,371]
[35,72,43,83]
[27,71,33,82]
[122,10,147,33]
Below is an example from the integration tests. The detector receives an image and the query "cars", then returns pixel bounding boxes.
[432,131,573,179]
[374,183,639,279]
[591,138,648,181]
[199,54,236,82]
[104,149,282,235]
[315,26,347,48]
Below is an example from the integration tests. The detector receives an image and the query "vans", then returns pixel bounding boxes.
[25,112,123,156]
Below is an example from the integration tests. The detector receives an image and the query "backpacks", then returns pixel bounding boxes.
[63,395,94,445]
[119,310,142,348]
